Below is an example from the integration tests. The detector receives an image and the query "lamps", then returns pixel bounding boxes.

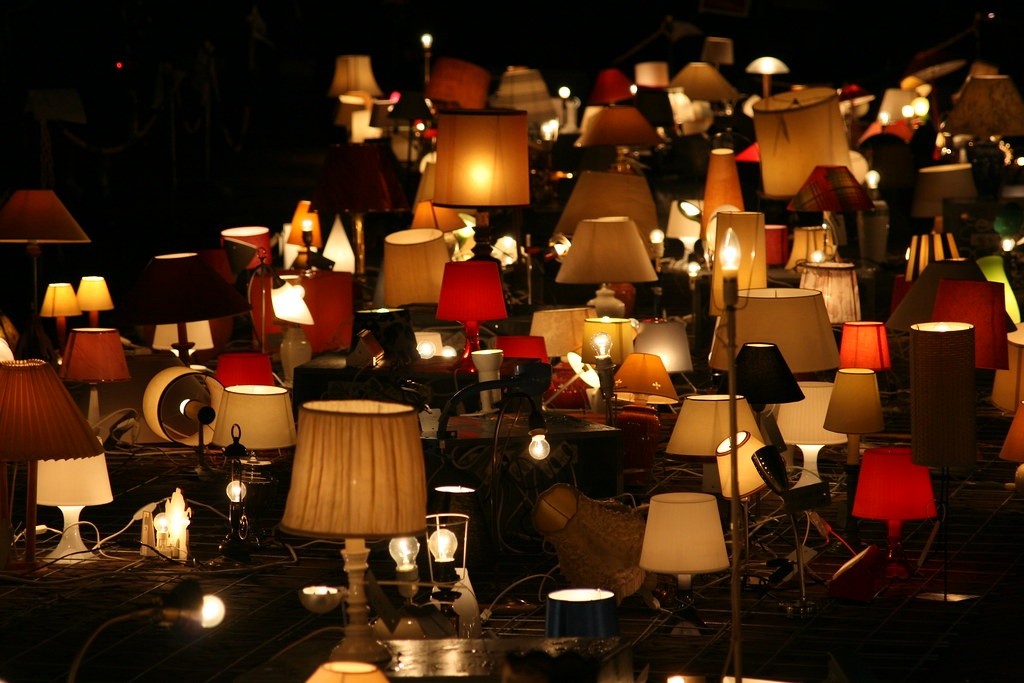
[0,33,1024,683]
